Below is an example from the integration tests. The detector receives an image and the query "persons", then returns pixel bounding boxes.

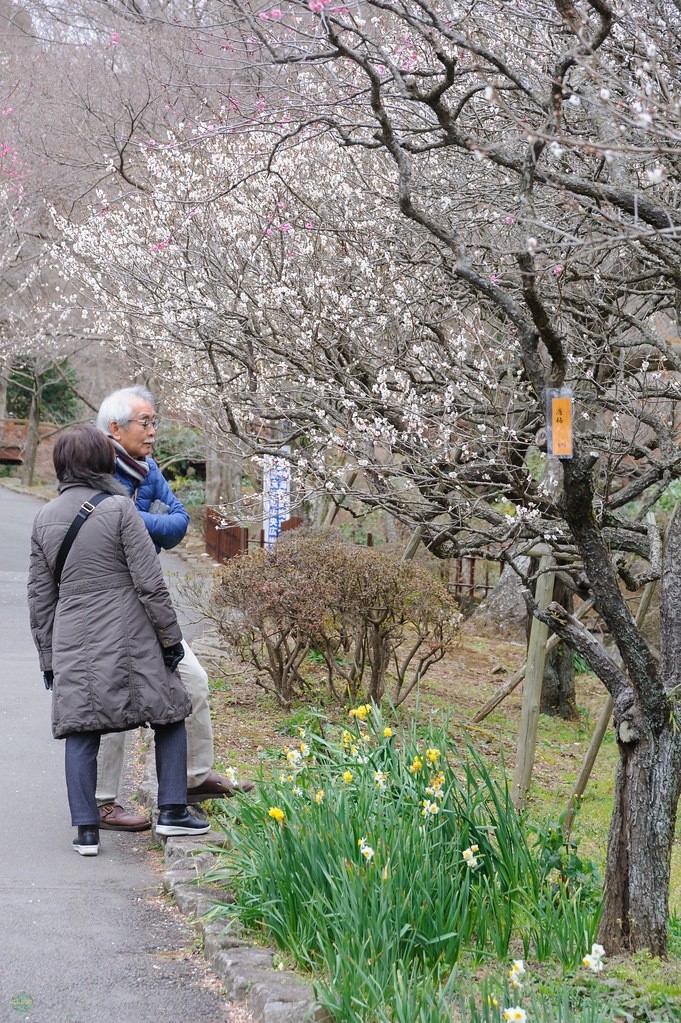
[25,424,213,855]
[94,385,258,834]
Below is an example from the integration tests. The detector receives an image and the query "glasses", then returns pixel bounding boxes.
[128,418,161,430]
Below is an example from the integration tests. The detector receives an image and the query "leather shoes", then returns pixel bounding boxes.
[185,771,254,805]
[95,801,152,832]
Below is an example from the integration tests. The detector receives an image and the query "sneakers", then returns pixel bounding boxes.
[72,831,101,855]
[156,806,212,836]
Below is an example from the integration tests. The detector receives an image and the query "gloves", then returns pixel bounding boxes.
[43,671,54,692]
[165,641,185,673]
[149,499,169,517]
